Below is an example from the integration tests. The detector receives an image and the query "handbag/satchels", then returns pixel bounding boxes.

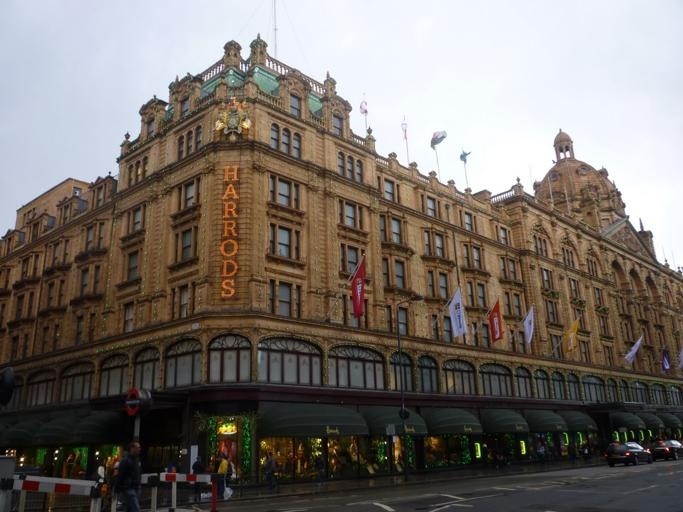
[90,486,99,498]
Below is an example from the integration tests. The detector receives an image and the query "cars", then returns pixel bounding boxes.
[603,439,683,467]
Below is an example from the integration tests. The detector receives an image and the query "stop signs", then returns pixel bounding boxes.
[125,389,140,416]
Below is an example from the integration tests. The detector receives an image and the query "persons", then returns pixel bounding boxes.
[192,456,204,502]
[581,439,592,465]
[262,459,280,495]
[97,441,143,512]
[213,453,232,502]
[570,440,578,463]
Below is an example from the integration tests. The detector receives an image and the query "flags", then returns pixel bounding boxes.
[430,128,448,150]
[522,305,534,347]
[448,284,467,337]
[351,254,365,320]
[624,336,644,365]
[566,319,578,354]
[359,100,370,115]
[489,297,504,345]
[662,349,671,370]
[460,151,470,162]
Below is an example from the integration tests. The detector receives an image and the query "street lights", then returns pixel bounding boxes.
[396,295,425,482]
